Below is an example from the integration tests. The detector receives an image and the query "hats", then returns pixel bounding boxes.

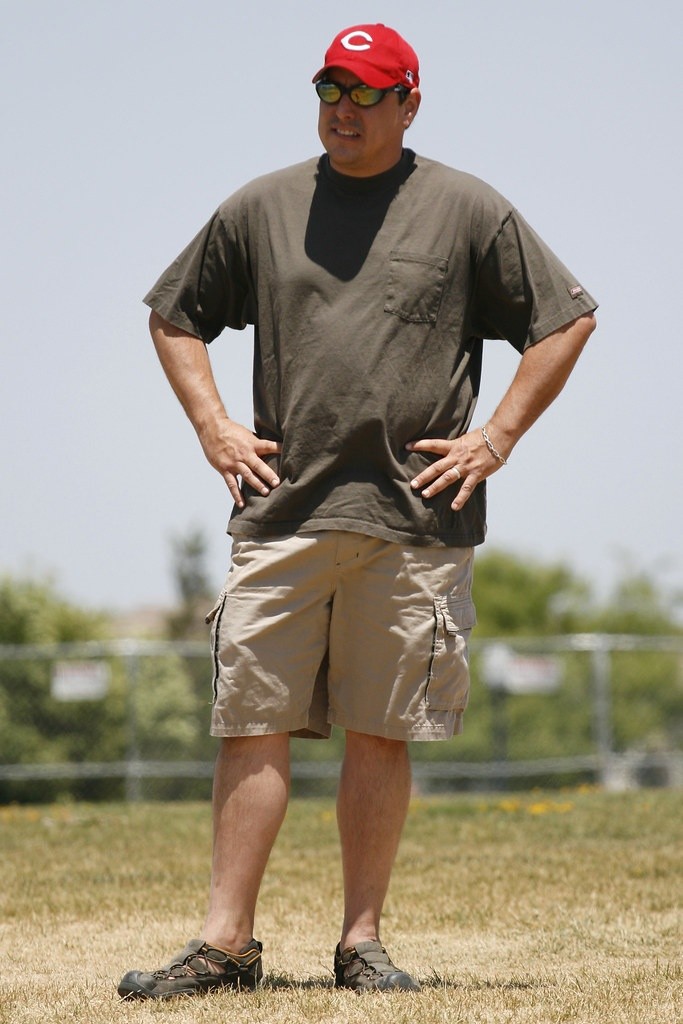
[312,23,420,90]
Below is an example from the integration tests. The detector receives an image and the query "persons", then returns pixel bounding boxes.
[118,25,601,999]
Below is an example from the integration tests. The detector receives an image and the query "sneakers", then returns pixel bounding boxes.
[118,937,263,1003]
[333,941,421,995]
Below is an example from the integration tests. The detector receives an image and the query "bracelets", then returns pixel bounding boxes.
[482,426,508,465]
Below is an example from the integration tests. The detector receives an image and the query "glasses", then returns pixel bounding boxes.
[316,80,409,107]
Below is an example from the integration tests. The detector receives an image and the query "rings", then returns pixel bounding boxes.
[452,467,461,479]
